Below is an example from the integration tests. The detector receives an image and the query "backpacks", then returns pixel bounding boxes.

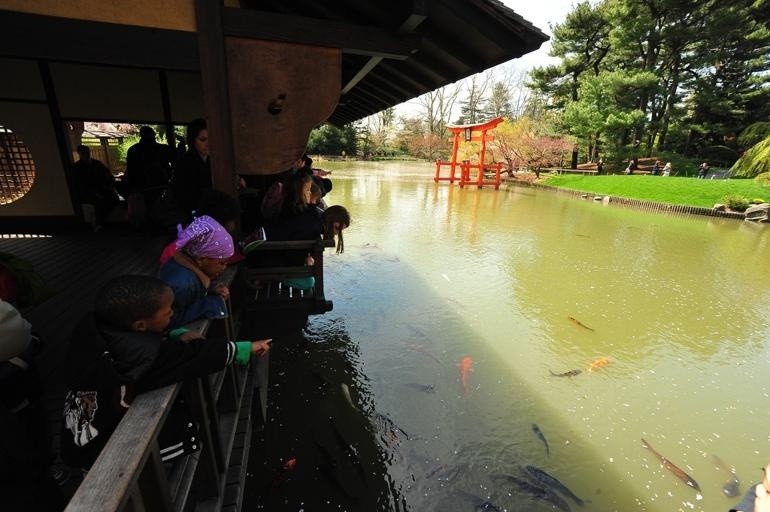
[261,180,284,217]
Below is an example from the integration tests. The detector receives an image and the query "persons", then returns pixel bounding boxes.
[697,162,711,179]
[90,275,273,510]
[161,190,245,266]
[75,145,115,232]
[2,334,68,510]
[127,119,212,235]
[572,143,578,169]
[238,155,349,291]
[626,159,635,175]
[597,158,604,173]
[652,160,659,175]
[160,217,234,329]
[663,162,671,176]
